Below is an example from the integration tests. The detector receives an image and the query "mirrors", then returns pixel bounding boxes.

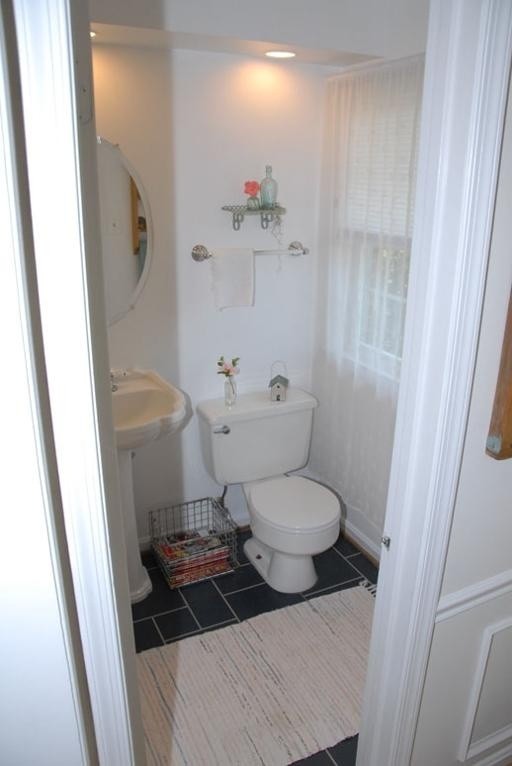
[93,134,154,326]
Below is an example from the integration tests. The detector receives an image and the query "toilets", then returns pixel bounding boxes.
[195,386,342,594]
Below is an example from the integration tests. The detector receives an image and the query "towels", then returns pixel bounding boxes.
[212,244,256,311]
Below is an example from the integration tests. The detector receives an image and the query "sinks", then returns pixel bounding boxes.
[108,368,188,450]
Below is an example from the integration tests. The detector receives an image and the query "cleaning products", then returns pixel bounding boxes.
[259,164,278,211]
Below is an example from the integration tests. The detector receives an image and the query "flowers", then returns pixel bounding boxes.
[218,354,239,393]
[243,179,260,205]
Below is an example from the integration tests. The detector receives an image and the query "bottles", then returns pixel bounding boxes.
[260,164,278,208]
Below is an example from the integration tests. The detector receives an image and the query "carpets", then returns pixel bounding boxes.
[138,580,382,764]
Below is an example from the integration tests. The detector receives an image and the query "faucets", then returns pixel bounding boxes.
[108,369,120,392]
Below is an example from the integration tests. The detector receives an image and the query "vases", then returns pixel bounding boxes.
[247,196,259,210]
[262,163,277,210]
[224,374,237,405]
